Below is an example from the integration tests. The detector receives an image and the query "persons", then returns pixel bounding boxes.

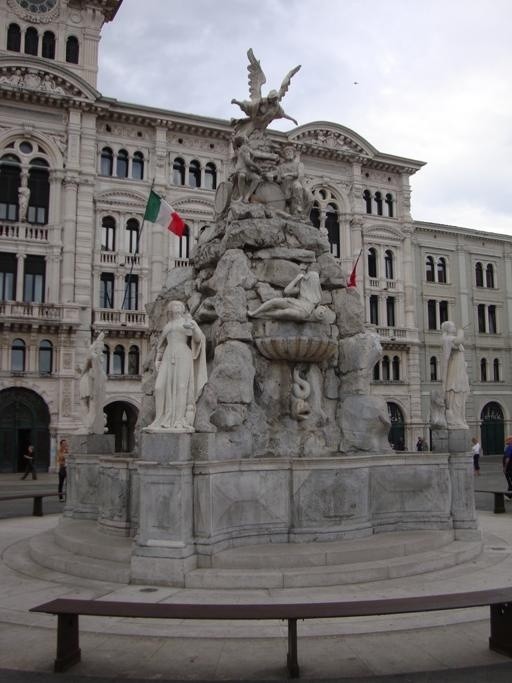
[416,436,423,450]
[502,435,512,501]
[40,75,66,95]
[247,270,324,320]
[1,69,24,84]
[471,436,481,477]
[21,445,38,480]
[440,319,470,427]
[230,89,308,215]
[421,437,429,451]
[72,338,108,434]
[57,439,70,503]
[147,298,209,429]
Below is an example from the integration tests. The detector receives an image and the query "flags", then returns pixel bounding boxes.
[144,190,184,237]
[347,266,356,288]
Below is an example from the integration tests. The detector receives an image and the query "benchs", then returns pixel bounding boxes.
[0,492,66,516]
[475,490,512,513]
[30,588,512,678]
